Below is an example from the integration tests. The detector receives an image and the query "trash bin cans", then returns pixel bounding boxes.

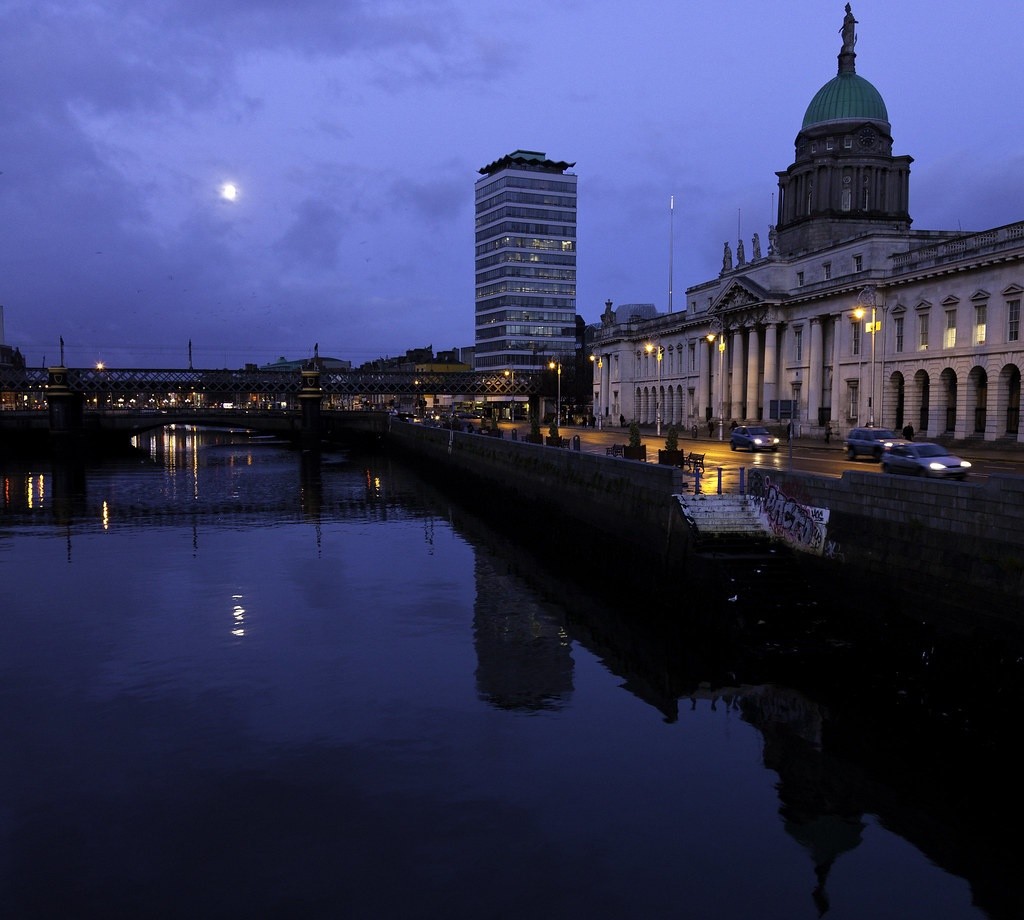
[691,425,697,438]
[572,435,580,450]
[511,429,517,441]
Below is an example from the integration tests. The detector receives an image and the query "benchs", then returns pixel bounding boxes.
[605,444,624,458]
[473,427,572,449]
[679,452,705,473]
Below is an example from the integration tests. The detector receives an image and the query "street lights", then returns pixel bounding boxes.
[854,287,877,427]
[644,332,661,436]
[504,362,514,423]
[706,319,724,441]
[590,345,603,432]
[415,377,423,418]
[668,194,673,316]
[549,354,561,427]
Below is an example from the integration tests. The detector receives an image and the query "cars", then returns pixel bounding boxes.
[881,442,973,479]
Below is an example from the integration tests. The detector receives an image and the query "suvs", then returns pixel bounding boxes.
[731,426,779,451]
[844,426,914,461]
